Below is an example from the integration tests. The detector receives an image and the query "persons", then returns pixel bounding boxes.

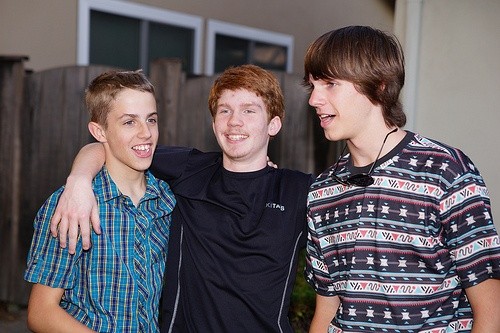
[24,69,278,333]
[298,24,500,333]
[50,63,320,333]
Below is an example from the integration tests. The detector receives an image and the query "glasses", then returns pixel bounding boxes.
[329,128,399,187]
